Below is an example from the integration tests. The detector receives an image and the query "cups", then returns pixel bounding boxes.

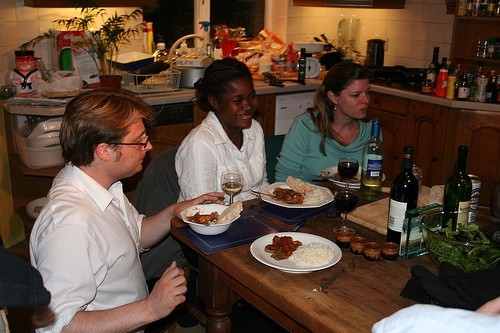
[382,242,399,262]
[362,242,382,261]
[349,235,367,254]
[335,228,355,249]
[412,164,424,184]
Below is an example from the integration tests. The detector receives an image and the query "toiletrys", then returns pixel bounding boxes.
[181,40,187,55]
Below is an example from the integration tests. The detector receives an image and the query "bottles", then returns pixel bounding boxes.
[385,146,419,244]
[14,50,48,82]
[419,0,499,104]
[440,144,473,231]
[142,20,306,85]
[360,118,384,201]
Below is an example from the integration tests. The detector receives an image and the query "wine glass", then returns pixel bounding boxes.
[337,157,359,190]
[333,189,360,234]
[221,169,244,206]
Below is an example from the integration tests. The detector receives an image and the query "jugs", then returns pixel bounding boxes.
[365,39,385,67]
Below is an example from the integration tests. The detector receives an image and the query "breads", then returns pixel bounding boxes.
[286,176,314,195]
[416,184,444,207]
[214,199,243,223]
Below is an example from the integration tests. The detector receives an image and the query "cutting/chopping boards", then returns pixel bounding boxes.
[341,196,389,235]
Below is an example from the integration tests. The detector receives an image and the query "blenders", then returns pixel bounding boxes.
[337,15,360,63]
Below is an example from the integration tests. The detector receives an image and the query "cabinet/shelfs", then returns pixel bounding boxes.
[366,93,500,212]
[449,0,500,65]
[0,101,76,244]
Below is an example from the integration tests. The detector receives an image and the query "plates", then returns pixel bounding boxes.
[27,196,46,219]
[259,181,335,208]
[250,232,342,274]
[321,166,386,188]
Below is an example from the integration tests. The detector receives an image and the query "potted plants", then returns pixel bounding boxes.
[16,4,145,92]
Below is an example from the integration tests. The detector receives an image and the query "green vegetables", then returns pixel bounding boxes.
[423,221,500,273]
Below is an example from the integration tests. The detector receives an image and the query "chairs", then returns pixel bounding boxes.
[0,134,288,333]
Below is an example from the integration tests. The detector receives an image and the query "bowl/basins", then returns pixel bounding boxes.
[180,203,241,236]
[14,117,65,170]
[420,211,500,272]
[172,66,206,87]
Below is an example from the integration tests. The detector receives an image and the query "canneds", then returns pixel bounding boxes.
[14,50,35,77]
[458,174,481,222]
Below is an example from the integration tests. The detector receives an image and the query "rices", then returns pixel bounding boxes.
[302,188,330,205]
[288,242,334,267]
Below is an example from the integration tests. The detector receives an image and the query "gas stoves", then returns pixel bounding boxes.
[367,65,428,91]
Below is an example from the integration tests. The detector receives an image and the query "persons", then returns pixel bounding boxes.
[28,88,225,333]
[9,50,43,94]
[275,61,383,184]
[175,57,270,270]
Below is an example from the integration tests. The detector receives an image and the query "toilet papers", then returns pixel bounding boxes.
[214,47,222,60]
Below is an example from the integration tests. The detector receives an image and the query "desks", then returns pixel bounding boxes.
[169,172,499,333]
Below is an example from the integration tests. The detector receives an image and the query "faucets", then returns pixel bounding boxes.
[153,49,178,62]
[168,34,204,66]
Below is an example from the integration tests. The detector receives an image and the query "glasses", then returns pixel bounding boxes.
[106,136,150,150]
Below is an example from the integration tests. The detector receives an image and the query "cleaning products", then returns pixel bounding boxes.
[199,21,213,58]
